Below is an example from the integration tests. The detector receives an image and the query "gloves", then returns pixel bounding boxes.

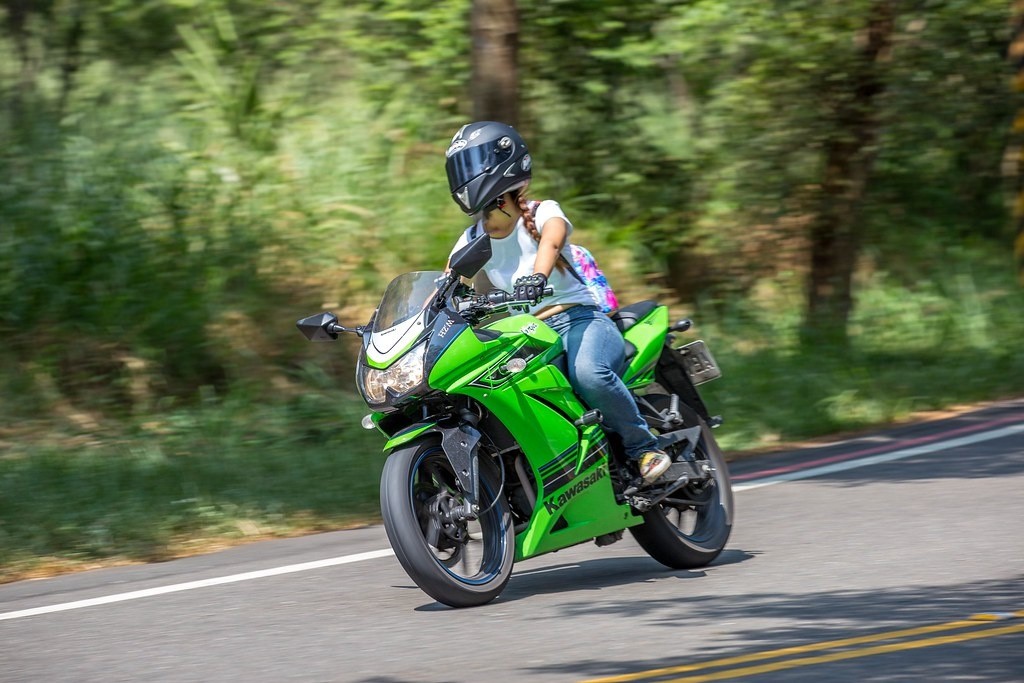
[510,273,547,313]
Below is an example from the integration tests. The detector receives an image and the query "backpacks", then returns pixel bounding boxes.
[470,199,619,316]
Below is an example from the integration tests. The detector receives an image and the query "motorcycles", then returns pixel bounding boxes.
[294,233,735,610]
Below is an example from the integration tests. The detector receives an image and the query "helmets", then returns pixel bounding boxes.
[445,121,532,215]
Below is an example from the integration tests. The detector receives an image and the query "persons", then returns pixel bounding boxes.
[424,119,674,484]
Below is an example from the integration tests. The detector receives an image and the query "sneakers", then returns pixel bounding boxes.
[637,448,672,482]
